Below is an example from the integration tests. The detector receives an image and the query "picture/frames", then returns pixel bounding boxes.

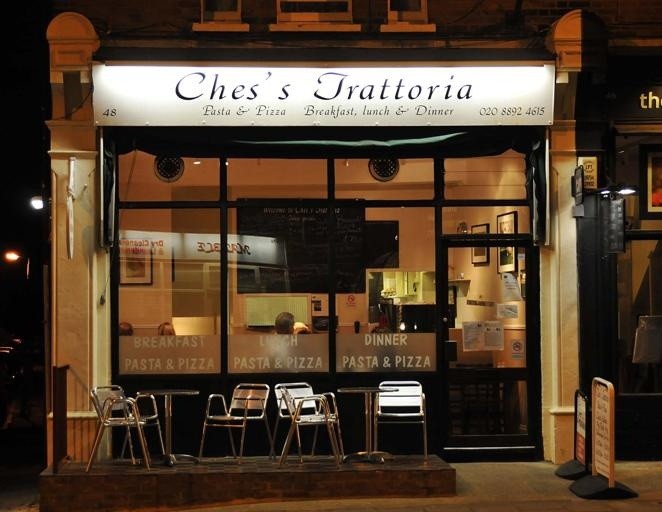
[638,143,662,220]
[470,223,492,267]
[171,247,175,282]
[117,237,153,285]
[496,210,518,274]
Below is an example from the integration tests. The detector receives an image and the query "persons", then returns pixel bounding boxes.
[118,311,413,376]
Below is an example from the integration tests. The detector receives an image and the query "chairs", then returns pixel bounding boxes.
[86,383,167,473]
[197,384,277,468]
[268,382,344,466]
[370,380,428,464]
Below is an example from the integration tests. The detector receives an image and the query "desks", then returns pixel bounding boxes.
[337,386,397,464]
[136,390,201,465]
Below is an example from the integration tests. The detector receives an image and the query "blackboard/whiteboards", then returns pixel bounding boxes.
[236,197,366,294]
[573,389,588,467]
[367,220,399,269]
[592,377,615,479]
